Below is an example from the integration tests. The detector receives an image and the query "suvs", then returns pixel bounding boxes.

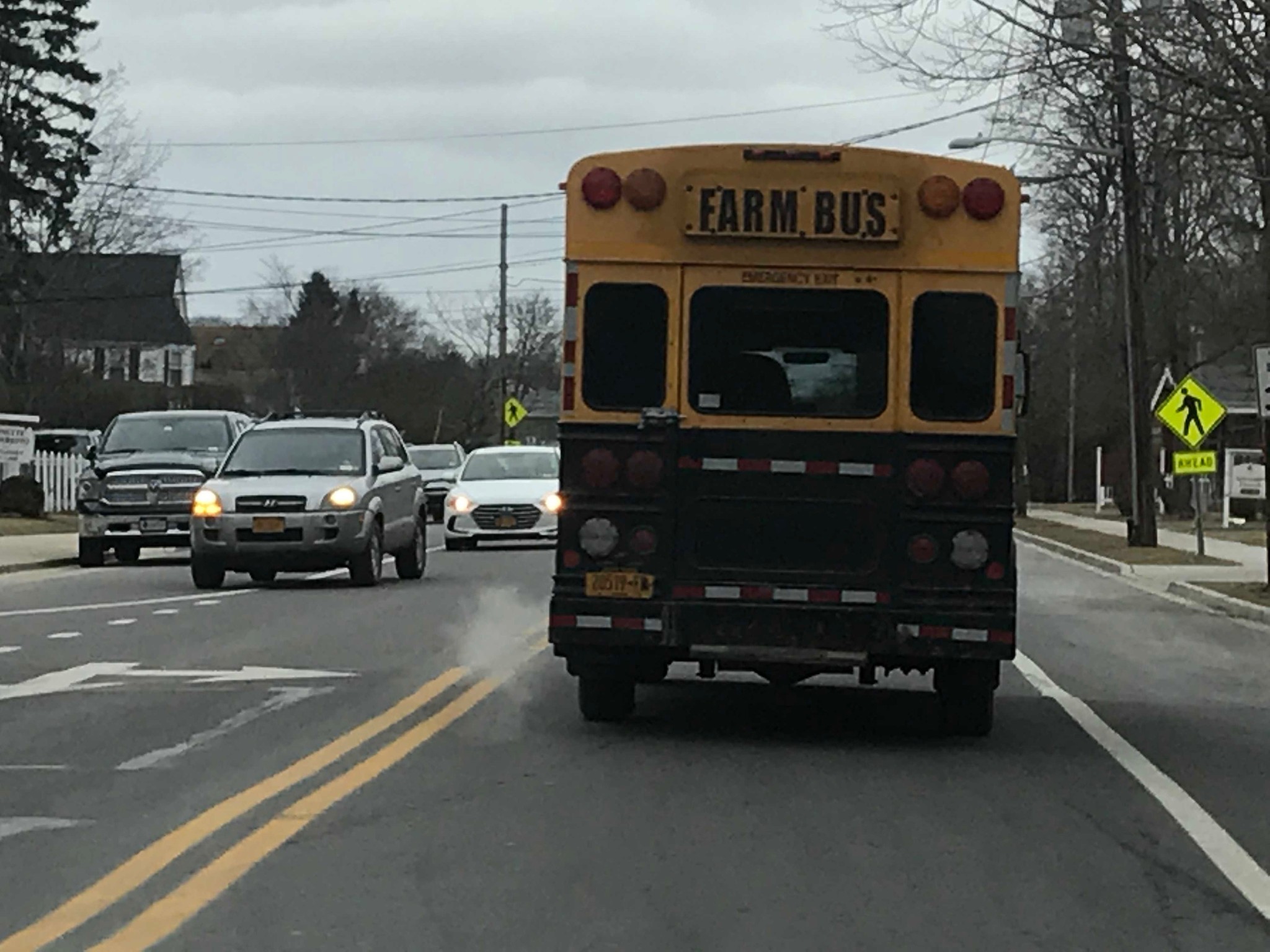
[188,408,429,589]
[401,440,468,521]
[73,409,254,567]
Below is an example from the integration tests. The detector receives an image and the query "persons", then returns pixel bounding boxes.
[535,457,555,475]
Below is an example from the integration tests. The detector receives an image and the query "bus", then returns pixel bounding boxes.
[546,141,1030,736]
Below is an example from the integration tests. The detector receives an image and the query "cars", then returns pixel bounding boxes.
[29,427,101,494]
[440,444,563,551]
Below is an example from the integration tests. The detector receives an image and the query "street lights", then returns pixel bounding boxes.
[945,131,1141,538]
